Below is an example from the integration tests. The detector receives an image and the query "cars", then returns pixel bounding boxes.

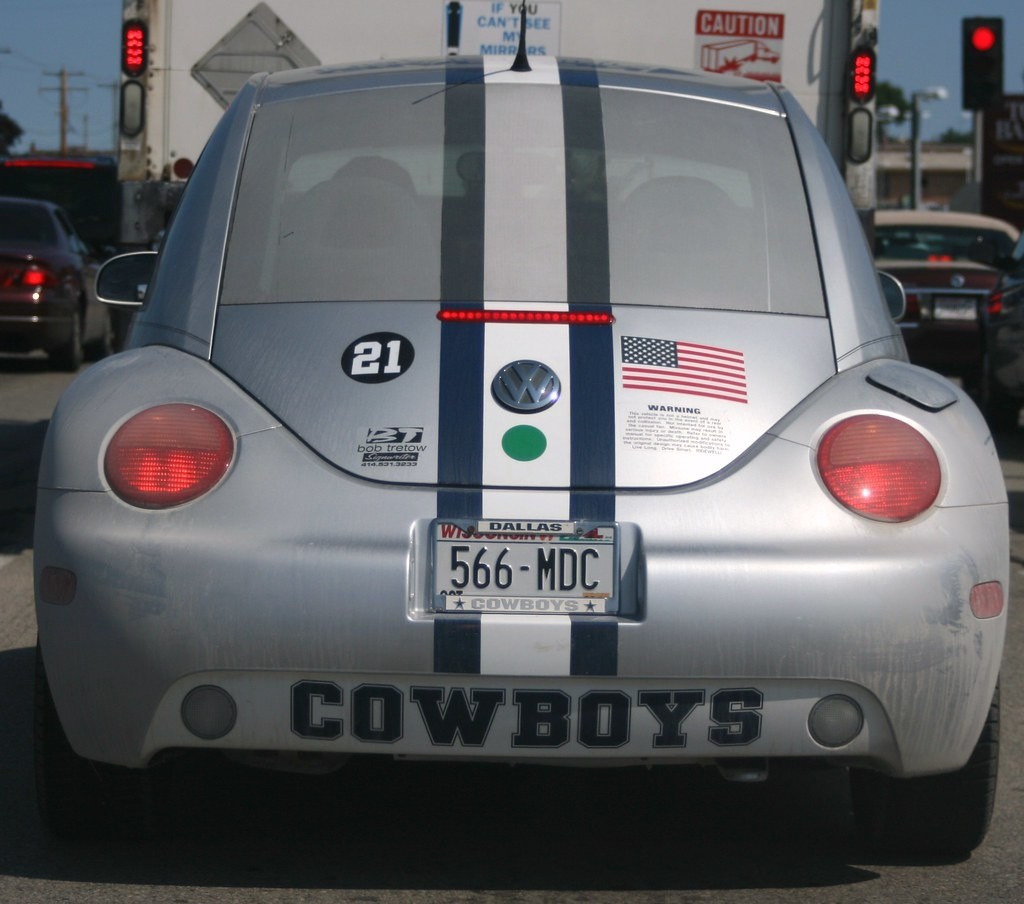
[32,51,1008,866]
[871,208,1021,453]
[0,198,96,370]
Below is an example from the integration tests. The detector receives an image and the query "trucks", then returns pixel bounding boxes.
[114,0,876,309]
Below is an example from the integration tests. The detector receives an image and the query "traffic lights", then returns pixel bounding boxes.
[960,14,1002,112]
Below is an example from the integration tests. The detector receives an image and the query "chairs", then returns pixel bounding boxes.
[611,175,768,310]
[264,175,439,300]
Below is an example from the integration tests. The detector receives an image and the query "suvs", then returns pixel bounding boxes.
[1,152,121,257]
